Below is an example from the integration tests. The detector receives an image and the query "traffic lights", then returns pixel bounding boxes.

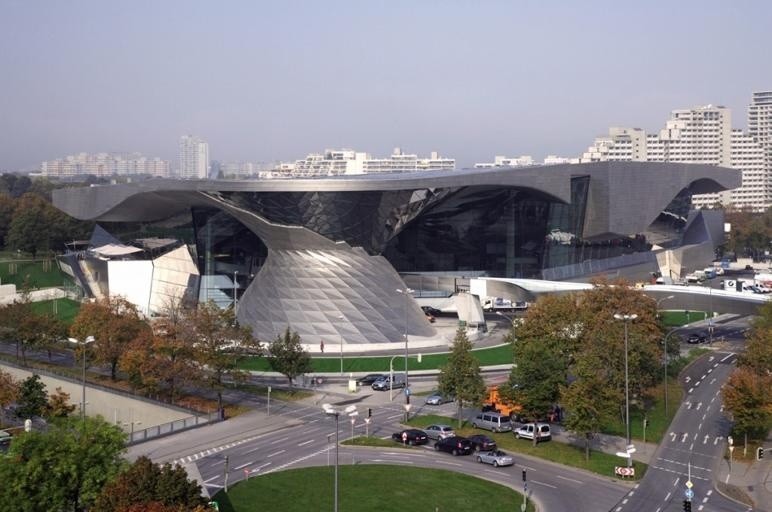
[367,408,373,416]
[687,501,691,511]
[757,446,763,461]
[682,500,687,511]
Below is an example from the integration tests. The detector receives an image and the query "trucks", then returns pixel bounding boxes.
[483,294,529,312]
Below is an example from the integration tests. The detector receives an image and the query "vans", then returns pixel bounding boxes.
[372,372,409,391]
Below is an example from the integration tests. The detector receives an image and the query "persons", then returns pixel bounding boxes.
[651,273,657,285]
[320,340,324,352]
[684,488,695,512]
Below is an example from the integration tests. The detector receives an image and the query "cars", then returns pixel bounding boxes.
[422,306,442,322]
[685,254,772,294]
[356,374,384,386]
[0,428,14,455]
[545,223,631,250]
[686,333,701,344]
[390,388,556,470]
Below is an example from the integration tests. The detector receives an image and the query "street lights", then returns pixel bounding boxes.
[613,311,639,469]
[641,293,675,322]
[232,270,239,326]
[321,403,359,512]
[497,310,517,366]
[68,335,95,422]
[338,315,345,373]
[696,280,726,326]
[663,324,689,414]
[395,288,415,389]
